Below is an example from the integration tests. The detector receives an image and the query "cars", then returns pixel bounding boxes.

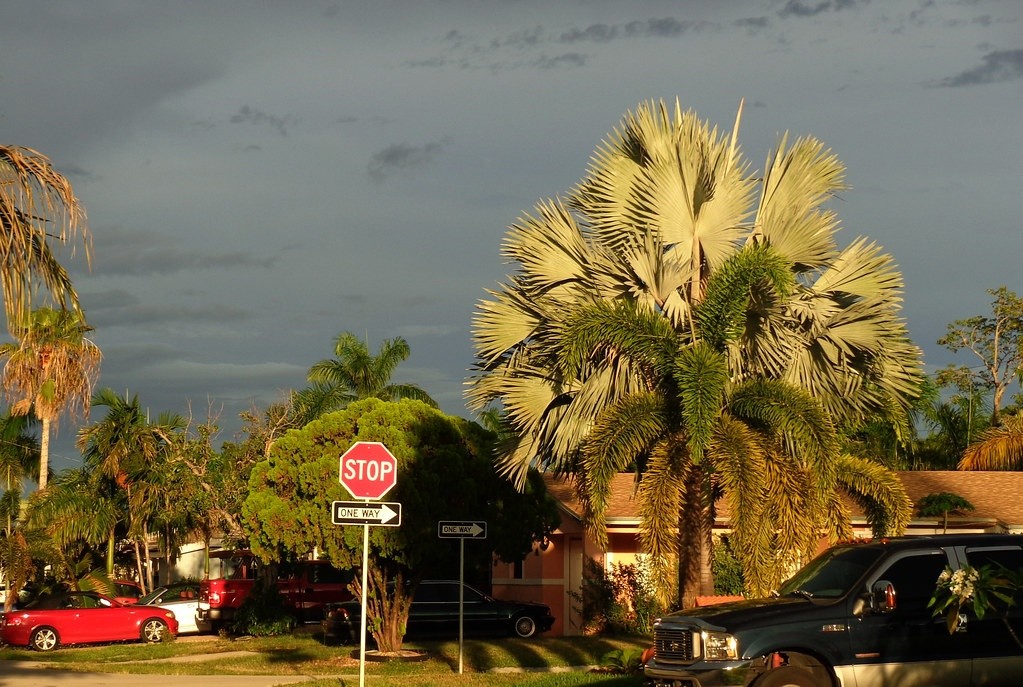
[134,583,199,636]
[323,579,555,643]
[0,591,179,651]
[109,582,151,605]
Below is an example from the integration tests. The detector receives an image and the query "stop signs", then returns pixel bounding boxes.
[339,442,397,501]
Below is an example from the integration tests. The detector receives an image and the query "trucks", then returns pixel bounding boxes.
[195,549,360,633]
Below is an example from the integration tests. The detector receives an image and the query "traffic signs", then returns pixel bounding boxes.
[331,501,401,527]
[438,521,486,539]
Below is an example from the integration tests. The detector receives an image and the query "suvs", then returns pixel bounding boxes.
[645,532,1023,687]
